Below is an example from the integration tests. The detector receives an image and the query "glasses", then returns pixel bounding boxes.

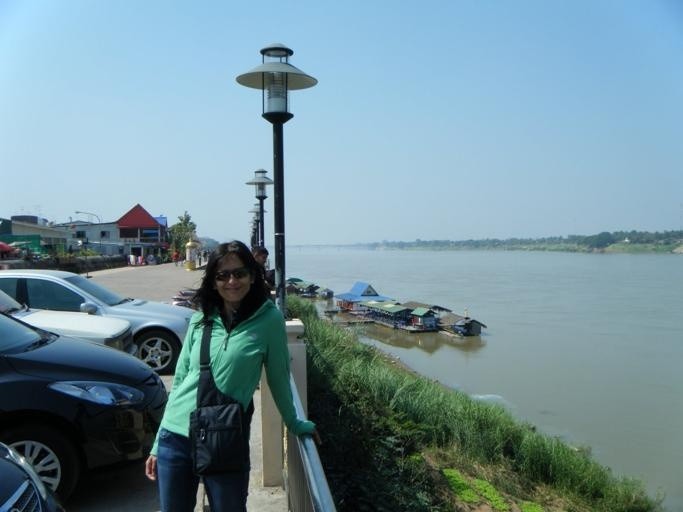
[213,265,250,281]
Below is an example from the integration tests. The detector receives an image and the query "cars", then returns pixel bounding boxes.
[0,442,69,512]
[0,289,140,374]
[0,268,200,373]
[0,313,170,506]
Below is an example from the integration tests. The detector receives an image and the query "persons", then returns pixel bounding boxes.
[251,247,269,264]
[146,240,322,512]
[172,250,179,267]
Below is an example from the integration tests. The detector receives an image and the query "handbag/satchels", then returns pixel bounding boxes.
[187,367,254,478]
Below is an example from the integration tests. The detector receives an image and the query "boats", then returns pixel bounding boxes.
[285,276,487,337]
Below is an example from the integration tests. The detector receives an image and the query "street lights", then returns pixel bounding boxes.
[75,210,104,256]
[249,204,269,254]
[234,42,324,322]
[247,168,276,259]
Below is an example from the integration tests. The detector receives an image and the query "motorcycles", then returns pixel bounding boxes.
[169,286,203,311]
